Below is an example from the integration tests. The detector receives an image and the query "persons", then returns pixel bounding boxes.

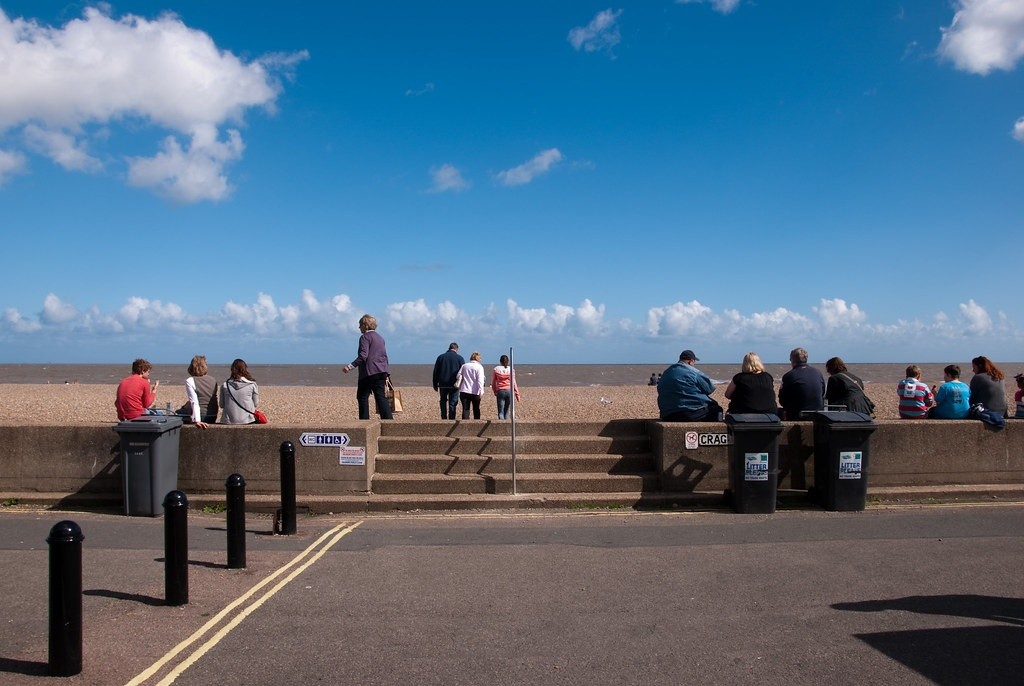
[219,359,259,425]
[432,342,465,420]
[491,355,520,419]
[897,364,933,419]
[648,373,656,386]
[453,352,484,419]
[342,313,394,419]
[657,373,662,384]
[175,355,219,429]
[656,350,724,422]
[724,352,778,415]
[778,347,826,420]
[65,381,70,385]
[825,357,876,419]
[1013,373,1024,419]
[926,365,971,419]
[969,356,1008,420]
[113,357,159,422]
[931,384,937,394]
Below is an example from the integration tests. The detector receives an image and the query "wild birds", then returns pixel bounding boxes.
[600,397,613,408]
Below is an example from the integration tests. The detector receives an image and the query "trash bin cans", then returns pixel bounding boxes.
[725,413,783,514]
[812,411,881,512]
[112,415,184,516]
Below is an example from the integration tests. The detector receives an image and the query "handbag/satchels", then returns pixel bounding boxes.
[254,409,268,424]
[454,375,463,388]
[865,394,876,409]
[376,375,404,413]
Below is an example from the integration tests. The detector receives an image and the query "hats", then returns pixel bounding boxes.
[679,350,699,362]
